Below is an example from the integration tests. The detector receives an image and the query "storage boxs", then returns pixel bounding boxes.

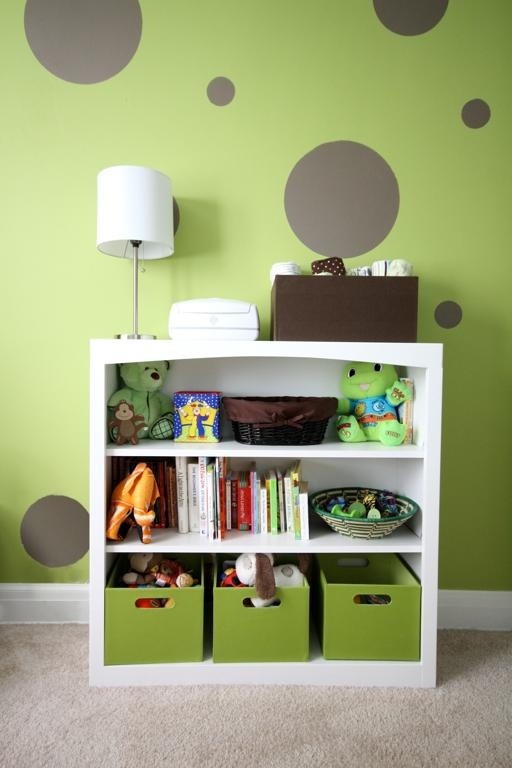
[269,273,419,343]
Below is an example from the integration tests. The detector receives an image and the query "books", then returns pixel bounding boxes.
[150,456,309,541]
[111,456,136,487]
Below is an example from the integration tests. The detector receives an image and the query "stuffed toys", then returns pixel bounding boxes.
[109,399,148,446]
[218,553,312,608]
[334,361,415,446]
[108,361,176,440]
[123,552,200,609]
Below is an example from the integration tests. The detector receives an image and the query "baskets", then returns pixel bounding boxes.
[222,391,339,445]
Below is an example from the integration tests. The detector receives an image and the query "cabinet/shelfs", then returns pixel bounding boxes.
[87,336,446,688]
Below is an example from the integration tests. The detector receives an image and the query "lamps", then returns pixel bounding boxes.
[96,162,179,340]
